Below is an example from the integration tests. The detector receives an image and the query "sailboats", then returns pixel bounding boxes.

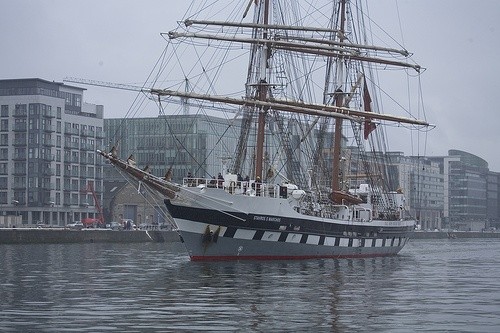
[96,0,436,262]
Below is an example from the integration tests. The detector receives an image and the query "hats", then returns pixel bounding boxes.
[256,176,260,179]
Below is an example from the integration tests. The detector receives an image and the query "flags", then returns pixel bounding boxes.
[364,82,376,139]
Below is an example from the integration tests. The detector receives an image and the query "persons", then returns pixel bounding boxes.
[124,220,131,230]
[238,174,262,196]
[188,172,224,188]
